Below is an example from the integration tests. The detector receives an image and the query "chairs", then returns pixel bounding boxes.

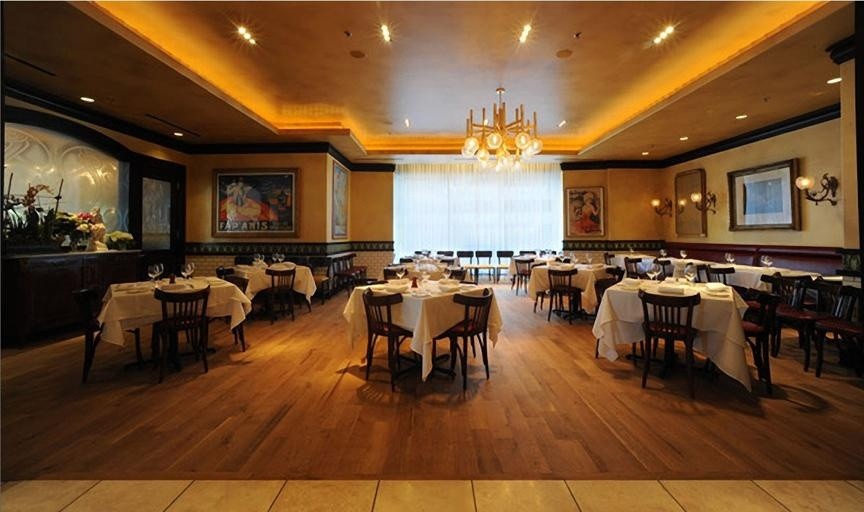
[216,266,235,280]
[415,251,431,255]
[438,251,454,265]
[813,285,864,379]
[309,256,333,304]
[540,251,556,256]
[532,263,550,313]
[260,267,296,324]
[475,250,495,284]
[363,287,422,391]
[153,284,211,385]
[708,265,735,285]
[457,251,474,280]
[746,275,810,355]
[776,276,842,371]
[436,287,494,391]
[497,251,516,282]
[638,288,701,400]
[201,276,248,352]
[71,288,142,384]
[654,258,671,279]
[515,259,534,295]
[520,251,536,256]
[623,257,643,278]
[548,269,584,324]
[709,289,780,395]
[332,254,368,290]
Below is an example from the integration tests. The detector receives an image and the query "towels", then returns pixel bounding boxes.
[624,277,640,286]
[439,286,458,292]
[117,283,135,288]
[658,283,685,294]
[665,277,676,281]
[706,281,728,293]
[162,277,182,281]
[238,264,250,270]
[678,279,688,284]
[388,279,408,284]
[162,284,186,289]
[439,279,460,284]
[209,278,224,285]
[386,286,409,293]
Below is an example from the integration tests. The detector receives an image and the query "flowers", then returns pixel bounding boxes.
[55,210,97,238]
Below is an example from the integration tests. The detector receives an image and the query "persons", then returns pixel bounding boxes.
[572,192,601,234]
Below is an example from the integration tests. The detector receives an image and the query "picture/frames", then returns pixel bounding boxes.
[566,186,608,239]
[332,160,349,239]
[674,168,707,237]
[726,157,802,232]
[211,167,303,238]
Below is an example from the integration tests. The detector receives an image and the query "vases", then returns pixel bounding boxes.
[69,238,90,252]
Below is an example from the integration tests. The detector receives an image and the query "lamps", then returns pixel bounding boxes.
[651,198,673,216]
[461,87,543,172]
[691,190,717,216]
[795,173,839,207]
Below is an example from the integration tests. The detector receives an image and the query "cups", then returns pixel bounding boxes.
[646,265,655,282]
[730,258,735,263]
[443,267,451,280]
[254,253,260,268]
[660,249,667,258]
[155,264,163,288]
[277,254,284,264]
[181,265,191,285]
[725,253,731,262]
[147,265,159,289]
[396,267,406,280]
[680,250,687,258]
[586,253,593,269]
[684,264,697,286]
[765,256,773,269]
[260,255,264,262]
[651,263,661,284]
[761,256,765,264]
[185,263,194,283]
[272,254,277,265]
[560,256,564,264]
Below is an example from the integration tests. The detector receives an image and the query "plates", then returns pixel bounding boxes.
[701,287,730,297]
